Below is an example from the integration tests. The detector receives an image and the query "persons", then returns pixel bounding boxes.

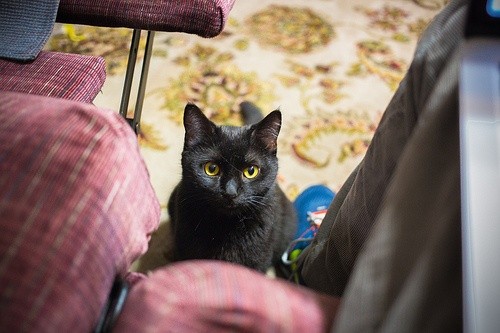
[281,0,500,333]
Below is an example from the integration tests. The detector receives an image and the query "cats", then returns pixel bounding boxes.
[167,100,296,278]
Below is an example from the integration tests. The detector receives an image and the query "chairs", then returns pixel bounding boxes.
[0,0,235,139]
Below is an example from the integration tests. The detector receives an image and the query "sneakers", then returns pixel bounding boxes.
[280,184,337,288]
